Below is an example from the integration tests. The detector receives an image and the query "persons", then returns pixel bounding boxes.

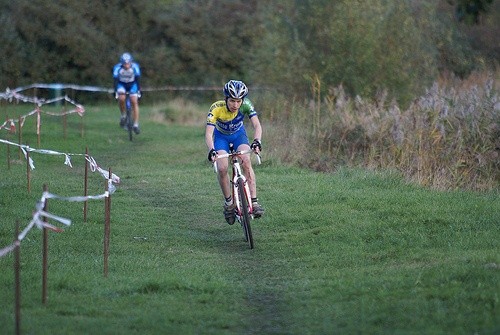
[113,53,142,135]
[205,80,264,225]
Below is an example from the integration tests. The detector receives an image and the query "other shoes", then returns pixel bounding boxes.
[133,127,140,134]
[120,117,127,126]
[224,204,235,226]
[253,204,264,215]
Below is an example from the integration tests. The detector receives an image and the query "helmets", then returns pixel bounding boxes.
[120,53,133,64]
[223,80,249,99]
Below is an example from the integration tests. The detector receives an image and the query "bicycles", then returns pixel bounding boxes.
[115,91,137,141]
[211,143,262,249]
